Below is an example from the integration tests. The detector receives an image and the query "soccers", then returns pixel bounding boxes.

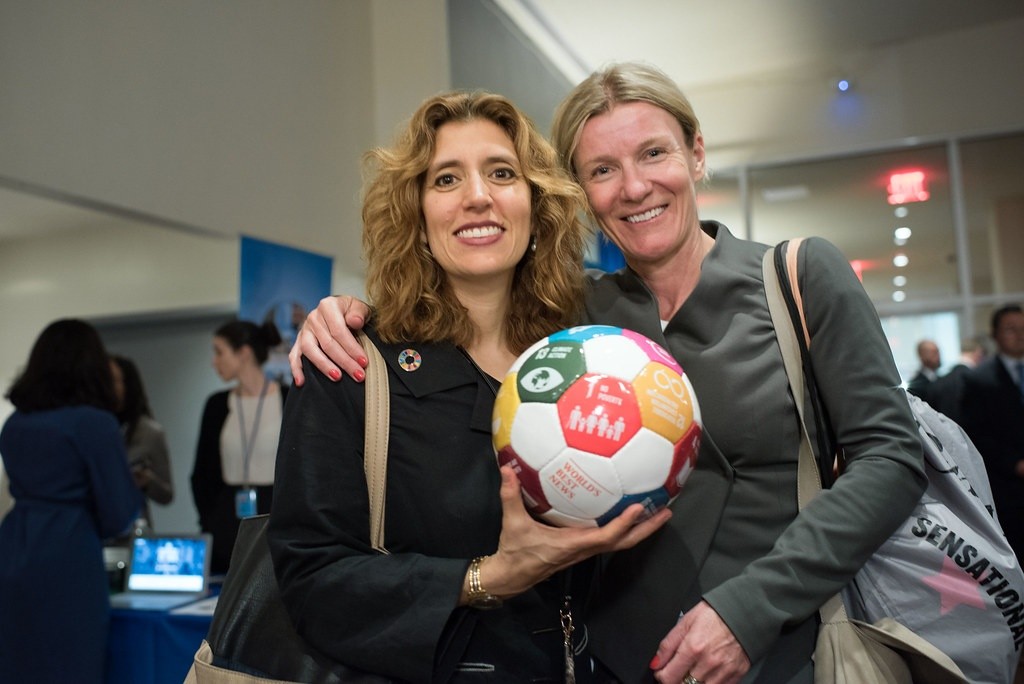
[492,324,702,526]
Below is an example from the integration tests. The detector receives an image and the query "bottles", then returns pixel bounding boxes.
[127,518,155,563]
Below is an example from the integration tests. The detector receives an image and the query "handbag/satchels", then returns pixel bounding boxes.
[763,236,1024,684]
[182,326,391,684]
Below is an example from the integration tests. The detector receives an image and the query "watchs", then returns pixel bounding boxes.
[467,555,508,612]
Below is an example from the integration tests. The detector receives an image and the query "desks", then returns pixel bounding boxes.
[107,585,221,684]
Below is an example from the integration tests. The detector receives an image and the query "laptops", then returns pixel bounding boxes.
[110,532,214,611]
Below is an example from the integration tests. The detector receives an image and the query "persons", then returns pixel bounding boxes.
[906,304,1024,569]
[266,90,673,684]
[103,349,173,535]
[190,318,291,578]
[1,318,142,684]
[288,60,925,684]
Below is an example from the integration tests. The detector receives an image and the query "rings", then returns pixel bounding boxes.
[682,669,705,684]
